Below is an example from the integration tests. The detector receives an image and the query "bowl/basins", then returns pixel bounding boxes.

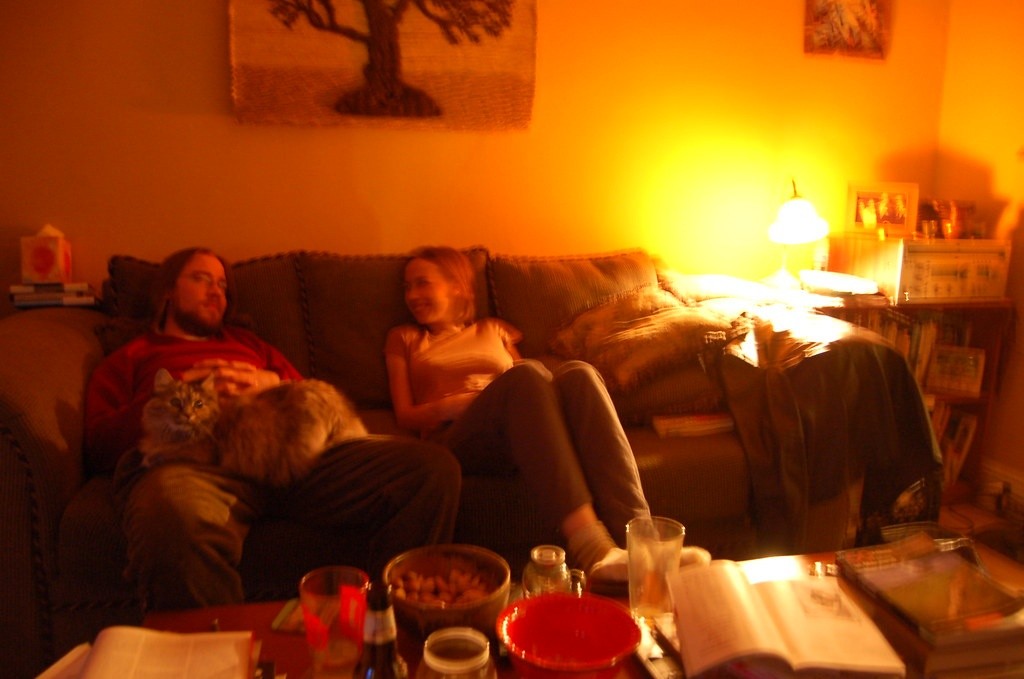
[383,543,511,642]
[495,592,642,679]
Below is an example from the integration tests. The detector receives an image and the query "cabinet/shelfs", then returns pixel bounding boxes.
[821,293,1016,505]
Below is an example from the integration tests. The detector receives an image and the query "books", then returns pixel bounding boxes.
[34,626,261,679]
[661,560,906,678]
[9,282,101,307]
[834,532,1024,679]
[841,309,978,487]
[651,412,734,438]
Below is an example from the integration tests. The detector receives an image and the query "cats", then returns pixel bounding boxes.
[136,368,369,487]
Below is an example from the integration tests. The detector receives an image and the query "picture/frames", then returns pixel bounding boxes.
[845,178,919,237]
[802,0,889,61]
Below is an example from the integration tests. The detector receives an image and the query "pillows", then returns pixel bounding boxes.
[94,317,155,356]
[553,285,748,419]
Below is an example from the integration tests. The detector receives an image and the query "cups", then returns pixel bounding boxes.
[626,515,686,642]
[922,197,986,240]
[298,564,369,679]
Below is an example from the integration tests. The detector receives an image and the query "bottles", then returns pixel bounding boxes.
[414,626,498,679]
[350,582,409,679]
[520,545,573,598]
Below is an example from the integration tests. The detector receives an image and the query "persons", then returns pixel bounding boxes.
[857,192,906,225]
[83,247,466,612]
[386,245,710,592]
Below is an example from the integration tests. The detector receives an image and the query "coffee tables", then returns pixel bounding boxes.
[141,541,1024,679]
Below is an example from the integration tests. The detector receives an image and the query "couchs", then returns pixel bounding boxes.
[0,244,944,679]
